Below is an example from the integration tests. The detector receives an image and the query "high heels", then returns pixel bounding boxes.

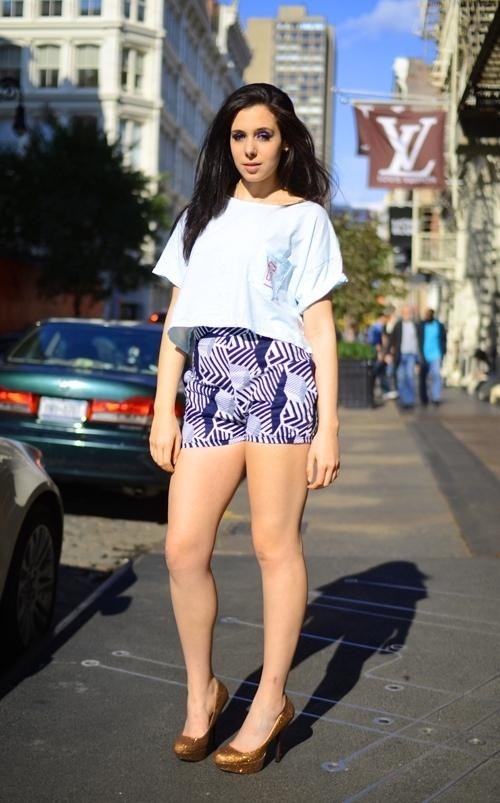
[213,690,297,774]
[173,672,229,763]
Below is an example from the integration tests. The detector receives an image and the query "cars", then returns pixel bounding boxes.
[0,437,68,671]
[146,309,170,322]
[0,316,190,505]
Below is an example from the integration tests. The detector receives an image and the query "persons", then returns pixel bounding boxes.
[362,304,448,412]
[147,81,351,775]
